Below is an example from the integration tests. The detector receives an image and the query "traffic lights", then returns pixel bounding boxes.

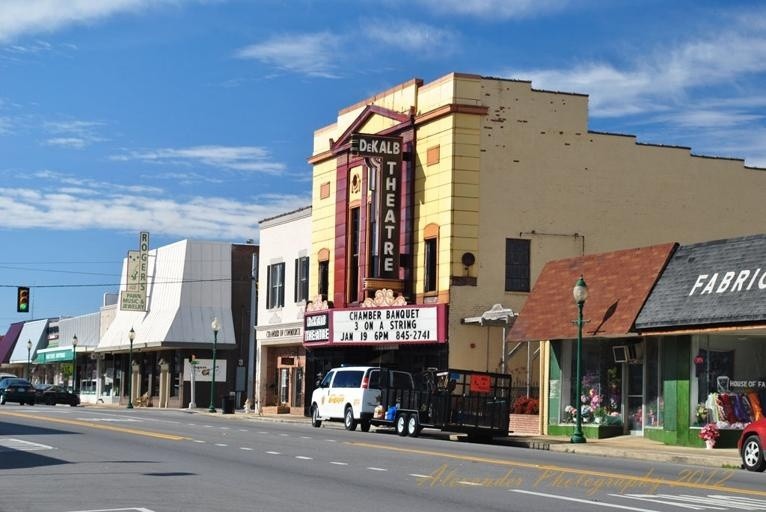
[17,287,29,313]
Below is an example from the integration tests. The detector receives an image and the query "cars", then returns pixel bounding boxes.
[738,417,766,471]
[0,373,80,406]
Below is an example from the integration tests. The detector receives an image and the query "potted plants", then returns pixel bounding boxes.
[593,406,604,424]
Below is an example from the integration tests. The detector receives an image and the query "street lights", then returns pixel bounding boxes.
[72,333,78,393]
[208,316,220,413]
[571,273,589,443]
[26,338,33,380]
[126,325,136,408]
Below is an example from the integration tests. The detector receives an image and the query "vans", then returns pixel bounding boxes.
[80,378,119,404]
[310,366,414,430]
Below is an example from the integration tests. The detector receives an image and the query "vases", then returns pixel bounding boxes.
[705,439,715,448]
[573,416,591,423]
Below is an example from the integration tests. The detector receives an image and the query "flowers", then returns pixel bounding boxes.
[698,421,721,446]
[564,387,603,416]
